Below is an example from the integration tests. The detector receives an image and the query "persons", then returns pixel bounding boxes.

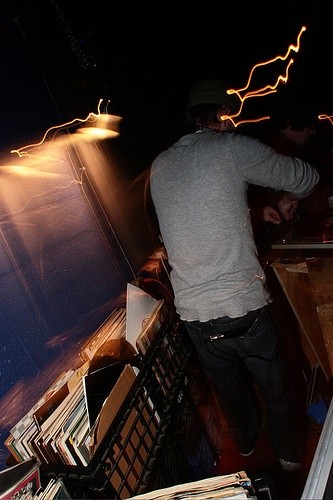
[148,82,321,473]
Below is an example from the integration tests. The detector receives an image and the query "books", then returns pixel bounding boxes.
[78,298,193,399]
[122,467,277,499]
[1,352,163,500]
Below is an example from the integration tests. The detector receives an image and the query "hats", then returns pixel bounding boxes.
[188,79,237,111]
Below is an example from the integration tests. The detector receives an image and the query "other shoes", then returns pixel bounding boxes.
[236,445,256,456]
[279,453,302,465]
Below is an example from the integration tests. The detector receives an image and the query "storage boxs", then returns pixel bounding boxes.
[6,307,189,500]
